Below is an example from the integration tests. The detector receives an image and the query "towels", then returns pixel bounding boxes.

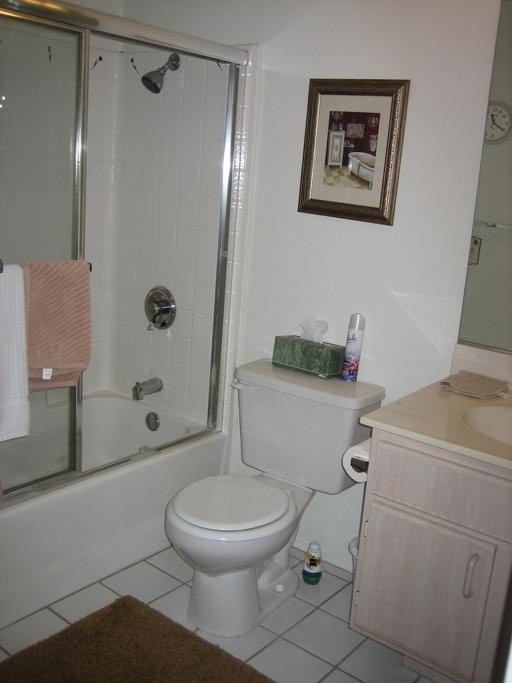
[1,262,30,441]
[23,258,94,389]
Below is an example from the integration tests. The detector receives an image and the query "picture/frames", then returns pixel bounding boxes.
[296,76,409,227]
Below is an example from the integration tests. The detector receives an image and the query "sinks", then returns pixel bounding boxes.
[460,402,512,447]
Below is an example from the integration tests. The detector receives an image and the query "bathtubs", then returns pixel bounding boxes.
[0,388,227,632]
[348,152,376,190]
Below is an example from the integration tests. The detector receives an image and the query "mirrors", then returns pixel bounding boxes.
[450,0,512,358]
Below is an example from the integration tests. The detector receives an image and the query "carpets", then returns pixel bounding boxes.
[1,595,277,682]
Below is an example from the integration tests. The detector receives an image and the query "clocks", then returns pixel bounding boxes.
[481,100,512,144]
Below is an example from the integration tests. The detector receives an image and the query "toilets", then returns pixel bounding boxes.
[164,359,386,637]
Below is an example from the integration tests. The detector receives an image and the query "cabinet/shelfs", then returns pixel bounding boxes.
[345,428,511,683]
